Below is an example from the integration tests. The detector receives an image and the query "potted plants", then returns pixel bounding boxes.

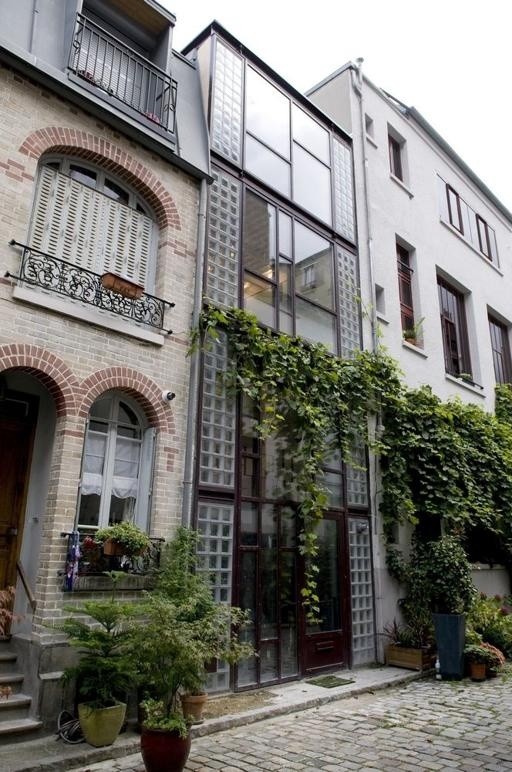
[368,536,505,680]
[40,517,259,772]
[403,317,425,345]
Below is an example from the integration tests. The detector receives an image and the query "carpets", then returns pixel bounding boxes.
[306,675,356,689]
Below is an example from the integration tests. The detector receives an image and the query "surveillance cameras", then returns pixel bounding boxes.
[162,390,175,402]
